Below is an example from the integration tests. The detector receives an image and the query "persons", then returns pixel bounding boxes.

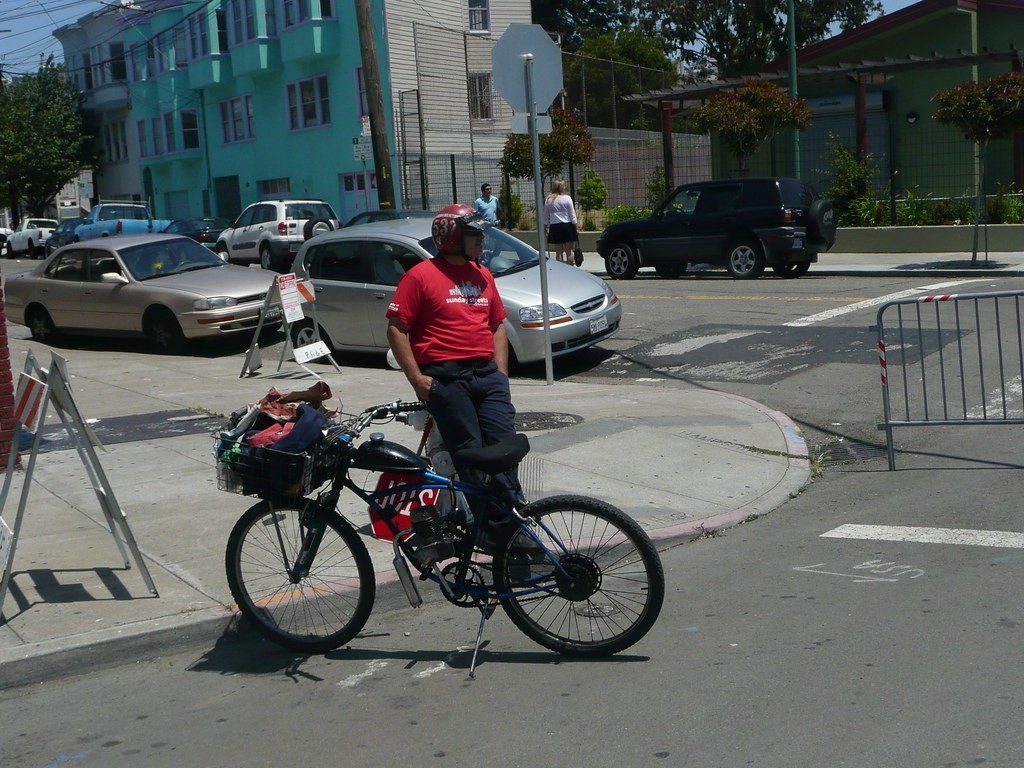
[543,179,579,265]
[472,184,506,229]
[386,204,539,552]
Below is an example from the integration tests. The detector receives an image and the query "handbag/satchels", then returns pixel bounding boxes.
[574,234,584,267]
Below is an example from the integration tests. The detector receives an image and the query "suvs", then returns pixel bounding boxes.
[215,198,346,275]
[599,177,838,281]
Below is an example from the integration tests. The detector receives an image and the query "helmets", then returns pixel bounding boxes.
[431,204,494,255]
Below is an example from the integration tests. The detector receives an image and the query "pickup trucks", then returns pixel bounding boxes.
[74,203,177,243]
[6,218,60,260]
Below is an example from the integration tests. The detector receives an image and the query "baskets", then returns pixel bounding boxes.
[209,405,361,503]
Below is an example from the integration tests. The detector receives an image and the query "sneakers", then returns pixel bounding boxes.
[486,530,539,551]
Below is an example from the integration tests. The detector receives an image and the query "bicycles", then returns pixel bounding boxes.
[212,395,667,677]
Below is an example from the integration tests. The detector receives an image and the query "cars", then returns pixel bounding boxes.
[42,215,88,262]
[0,227,13,255]
[285,216,622,377]
[339,209,499,231]
[154,216,235,260]
[5,232,293,353]
